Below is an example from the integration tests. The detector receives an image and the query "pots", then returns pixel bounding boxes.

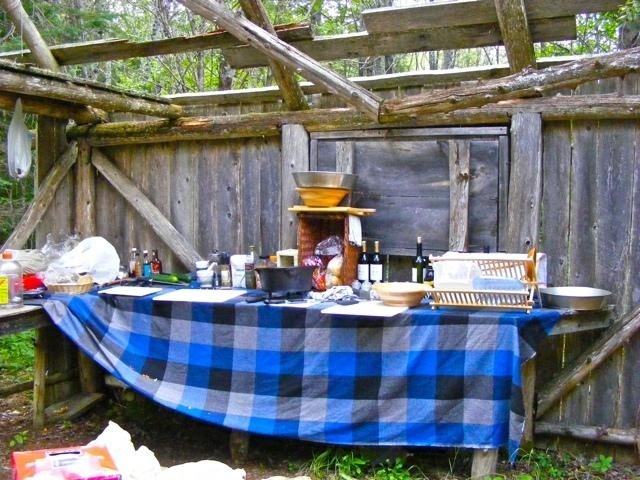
[255,265,319,302]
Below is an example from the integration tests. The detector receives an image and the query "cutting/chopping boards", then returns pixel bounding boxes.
[288,204,376,217]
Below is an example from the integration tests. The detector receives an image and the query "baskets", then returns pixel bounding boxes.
[45,274,97,295]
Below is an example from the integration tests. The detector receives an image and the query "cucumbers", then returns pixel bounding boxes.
[148,274,178,283]
[169,272,191,282]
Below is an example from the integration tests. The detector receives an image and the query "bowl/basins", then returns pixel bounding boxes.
[374,282,431,306]
[291,171,360,190]
[539,286,612,311]
[296,187,349,208]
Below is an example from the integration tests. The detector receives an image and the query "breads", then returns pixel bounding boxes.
[324,257,343,289]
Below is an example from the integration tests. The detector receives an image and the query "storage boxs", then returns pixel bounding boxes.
[10,446,123,480]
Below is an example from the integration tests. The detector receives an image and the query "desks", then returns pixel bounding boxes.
[0,283,616,480]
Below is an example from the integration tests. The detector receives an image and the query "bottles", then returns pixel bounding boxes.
[411,236,427,282]
[129,248,161,277]
[383,254,392,282]
[357,241,382,284]
[245,245,260,289]
